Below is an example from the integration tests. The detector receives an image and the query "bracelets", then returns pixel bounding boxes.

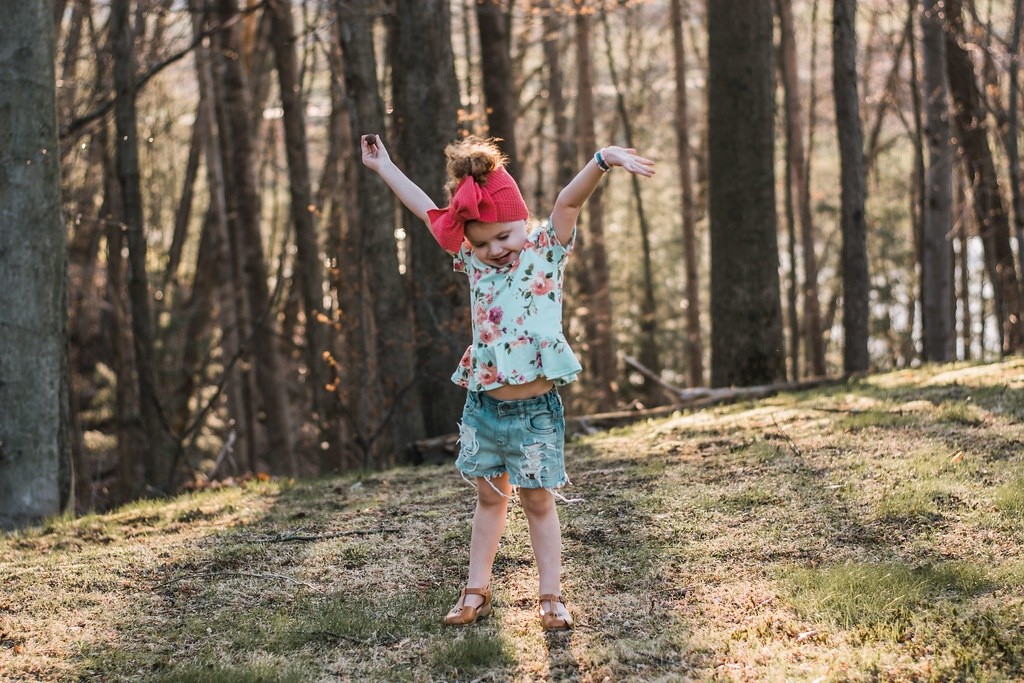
[594,148,611,172]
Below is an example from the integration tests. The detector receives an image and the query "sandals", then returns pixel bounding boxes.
[442,583,493,626]
[538,594,574,631]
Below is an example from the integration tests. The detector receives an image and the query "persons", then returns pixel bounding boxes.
[360,132,655,629]
